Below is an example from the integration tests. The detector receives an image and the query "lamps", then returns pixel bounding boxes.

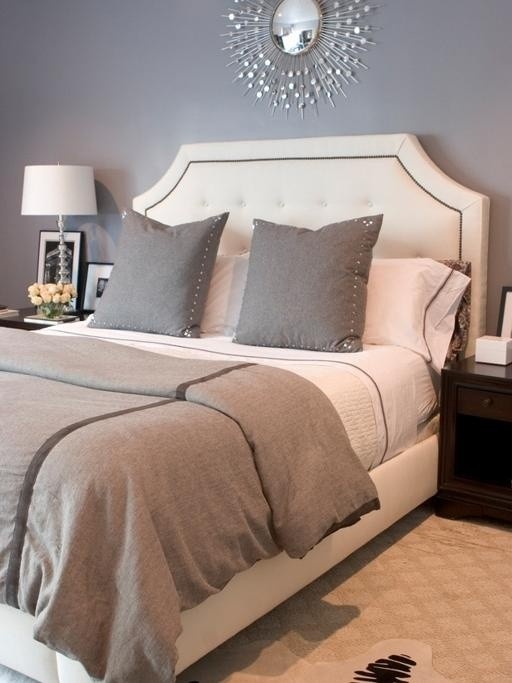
[20,165,98,326]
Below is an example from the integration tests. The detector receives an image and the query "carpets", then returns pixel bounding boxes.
[175,638,458,683]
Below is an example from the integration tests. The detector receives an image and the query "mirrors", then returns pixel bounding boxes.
[269,0,321,56]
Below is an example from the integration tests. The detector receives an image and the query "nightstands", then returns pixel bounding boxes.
[437,354,512,521]
[0,306,92,331]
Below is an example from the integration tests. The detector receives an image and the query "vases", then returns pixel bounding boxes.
[36,302,67,320]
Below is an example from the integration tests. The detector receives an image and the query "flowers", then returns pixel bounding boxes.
[27,283,79,319]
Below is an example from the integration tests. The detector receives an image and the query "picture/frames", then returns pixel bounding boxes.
[37,230,83,319]
[496,287,512,338]
[81,262,115,314]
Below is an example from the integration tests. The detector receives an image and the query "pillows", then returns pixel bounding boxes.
[84,251,250,338]
[231,214,383,353]
[87,208,236,338]
[222,255,471,376]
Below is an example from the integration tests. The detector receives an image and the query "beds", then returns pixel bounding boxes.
[1,133,491,683]
